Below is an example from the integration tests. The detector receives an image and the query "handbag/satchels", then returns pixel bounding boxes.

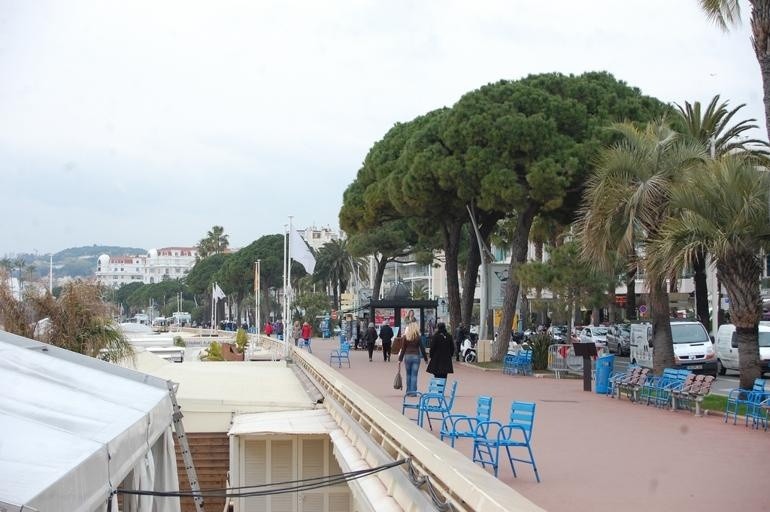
[394,372,402,389]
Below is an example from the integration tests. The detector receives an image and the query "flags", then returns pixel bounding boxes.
[216,285,226,299]
[291,223,316,274]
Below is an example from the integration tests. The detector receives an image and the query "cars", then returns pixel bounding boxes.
[521,320,770,382]
[127,308,191,326]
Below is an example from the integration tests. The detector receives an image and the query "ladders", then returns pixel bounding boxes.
[166,379,204,512]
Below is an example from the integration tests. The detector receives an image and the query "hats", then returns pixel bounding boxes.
[384,321,388,325]
[369,323,374,327]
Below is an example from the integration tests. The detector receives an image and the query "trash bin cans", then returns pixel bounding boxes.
[595,354,615,394]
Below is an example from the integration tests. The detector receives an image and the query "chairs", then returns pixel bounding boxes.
[402,378,458,440]
[473,400,540,484]
[502,348,533,375]
[440,396,496,470]
[606,362,770,432]
[329,343,351,369]
[299,337,312,354]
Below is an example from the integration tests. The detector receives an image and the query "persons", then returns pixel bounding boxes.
[159,317,283,341]
[397,321,428,397]
[362,322,378,361]
[292,320,300,346]
[379,320,393,362]
[453,322,466,362]
[300,321,312,353]
[404,310,416,324]
[425,322,455,393]
[507,336,525,373]
